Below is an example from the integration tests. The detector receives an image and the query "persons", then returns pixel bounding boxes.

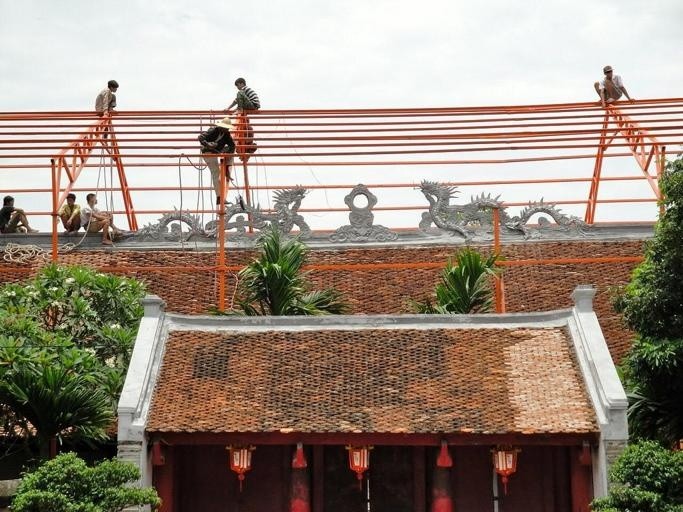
[222,76,261,114]
[56,193,81,235]
[79,193,125,244]
[198,117,237,205]
[235,117,259,162]
[94,80,119,143]
[593,65,635,108]
[0,195,41,234]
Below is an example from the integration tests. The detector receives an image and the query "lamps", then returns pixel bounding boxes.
[490,442,522,496]
[345,444,375,491]
[227,442,256,493]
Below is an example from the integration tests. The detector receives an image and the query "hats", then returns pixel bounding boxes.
[214,117,236,129]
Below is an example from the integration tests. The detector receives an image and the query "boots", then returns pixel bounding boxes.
[216,197,229,204]
